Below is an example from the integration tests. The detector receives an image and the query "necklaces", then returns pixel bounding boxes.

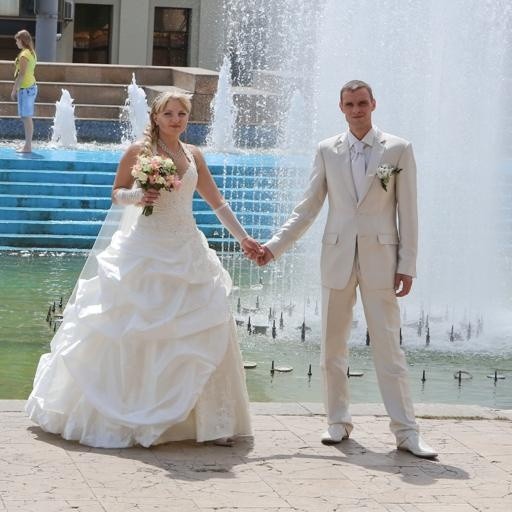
[156,138,184,162]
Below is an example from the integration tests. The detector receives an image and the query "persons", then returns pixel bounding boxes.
[10,29,39,153]
[22,91,264,450]
[244,79,439,458]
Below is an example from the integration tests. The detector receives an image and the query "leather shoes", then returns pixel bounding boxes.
[321,426,349,445]
[397,436,439,458]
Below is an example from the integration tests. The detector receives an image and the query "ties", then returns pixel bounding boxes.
[350,142,367,202]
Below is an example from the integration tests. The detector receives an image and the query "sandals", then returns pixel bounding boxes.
[203,438,236,447]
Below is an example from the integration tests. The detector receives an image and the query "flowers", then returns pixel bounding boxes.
[376,163,403,192]
[131,154,183,217]
